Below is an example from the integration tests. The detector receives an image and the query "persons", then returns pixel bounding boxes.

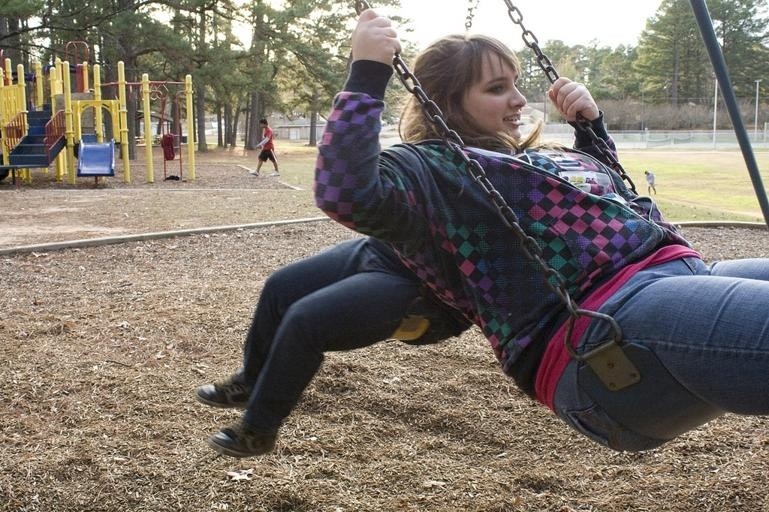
[249,120,282,177]
[644,167,657,199]
[192,233,468,459]
[312,6,767,451]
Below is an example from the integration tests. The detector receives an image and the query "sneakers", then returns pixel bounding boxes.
[271,171,281,176]
[250,171,259,176]
[208,422,279,458]
[194,366,256,409]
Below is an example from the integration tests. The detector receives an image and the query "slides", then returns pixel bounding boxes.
[77,139,116,177]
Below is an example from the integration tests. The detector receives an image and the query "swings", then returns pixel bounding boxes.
[377,0,485,347]
[354,1,731,452]
